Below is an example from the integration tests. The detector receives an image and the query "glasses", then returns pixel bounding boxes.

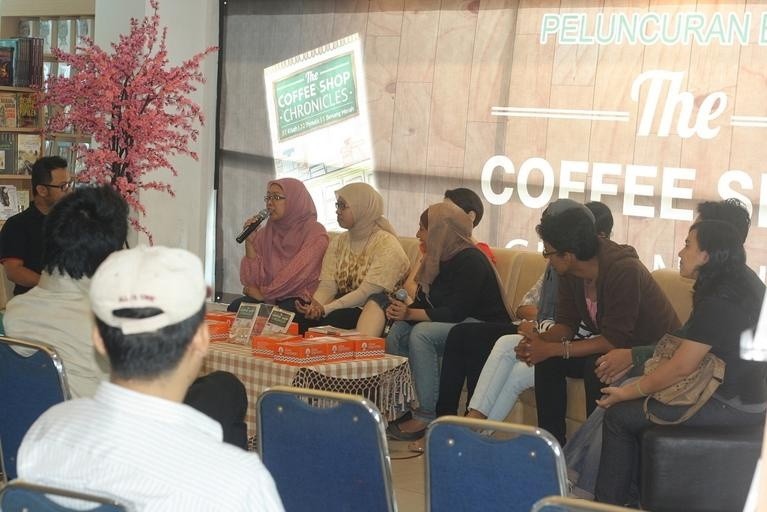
[37,176,75,192]
[264,196,286,202]
[542,250,563,258]
[335,203,350,210]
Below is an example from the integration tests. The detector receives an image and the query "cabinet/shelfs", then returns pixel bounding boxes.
[0,86,47,228]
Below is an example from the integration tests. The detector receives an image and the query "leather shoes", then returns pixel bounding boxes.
[385,408,416,437]
[388,418,426,441]
[408,436,426,453]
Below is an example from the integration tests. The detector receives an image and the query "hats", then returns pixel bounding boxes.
[88,245,208,336]
[445,187,483,226]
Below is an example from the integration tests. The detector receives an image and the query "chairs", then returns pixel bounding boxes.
[254,386,396,512]
[423,415,570,512]
[0,334,70,482]
[0,482,134,512]
[529,495,643,511]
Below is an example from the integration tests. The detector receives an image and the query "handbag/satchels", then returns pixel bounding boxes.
[643,332,727,426]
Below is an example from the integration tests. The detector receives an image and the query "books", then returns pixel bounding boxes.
[229,302,261,344]
[308,324,360,337]
[1,34,44,222]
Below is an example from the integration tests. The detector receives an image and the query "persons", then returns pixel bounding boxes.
[2,185,129,402]
[593,221,767,508]
[515,198,682,449]
[560,197,752,503]
[227,178,328,324]
[293,182,411,337]
[464,200,614,423]
[385,202,518,442]
[402,186,497,304]
[17,241,286,512]
[0,156,73,296]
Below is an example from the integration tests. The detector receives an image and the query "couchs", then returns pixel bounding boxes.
[324,231,697,445]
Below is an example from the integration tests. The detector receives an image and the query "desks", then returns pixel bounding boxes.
[198,336,421,453]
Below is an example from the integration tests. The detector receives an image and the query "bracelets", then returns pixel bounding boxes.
[561,336,570,361]
[636,380,648,397]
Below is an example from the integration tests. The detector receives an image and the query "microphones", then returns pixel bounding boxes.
[236,208,270,244]
[382,288,409,336]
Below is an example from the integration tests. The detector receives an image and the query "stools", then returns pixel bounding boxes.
[638,423,762,511]
